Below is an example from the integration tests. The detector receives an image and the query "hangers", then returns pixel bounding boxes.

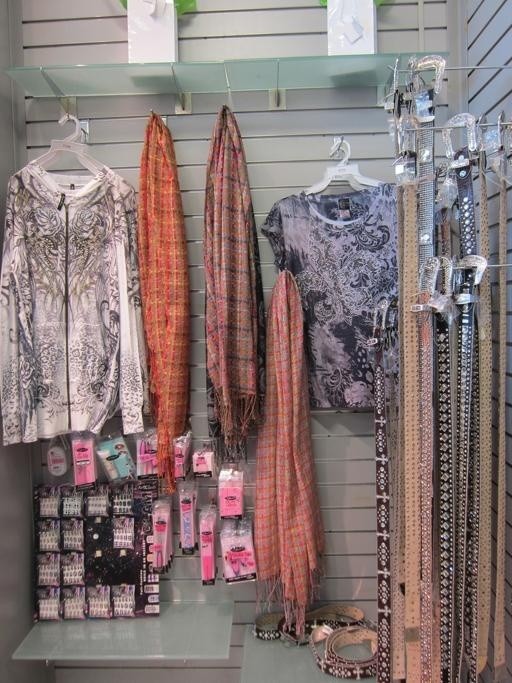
[309,150,369,192]
[35,115,105,180]
[306,141,385,192]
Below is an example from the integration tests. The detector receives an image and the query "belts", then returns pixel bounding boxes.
[306,619,379,679]
[375,58,508,683]
[250,601,364,648]
